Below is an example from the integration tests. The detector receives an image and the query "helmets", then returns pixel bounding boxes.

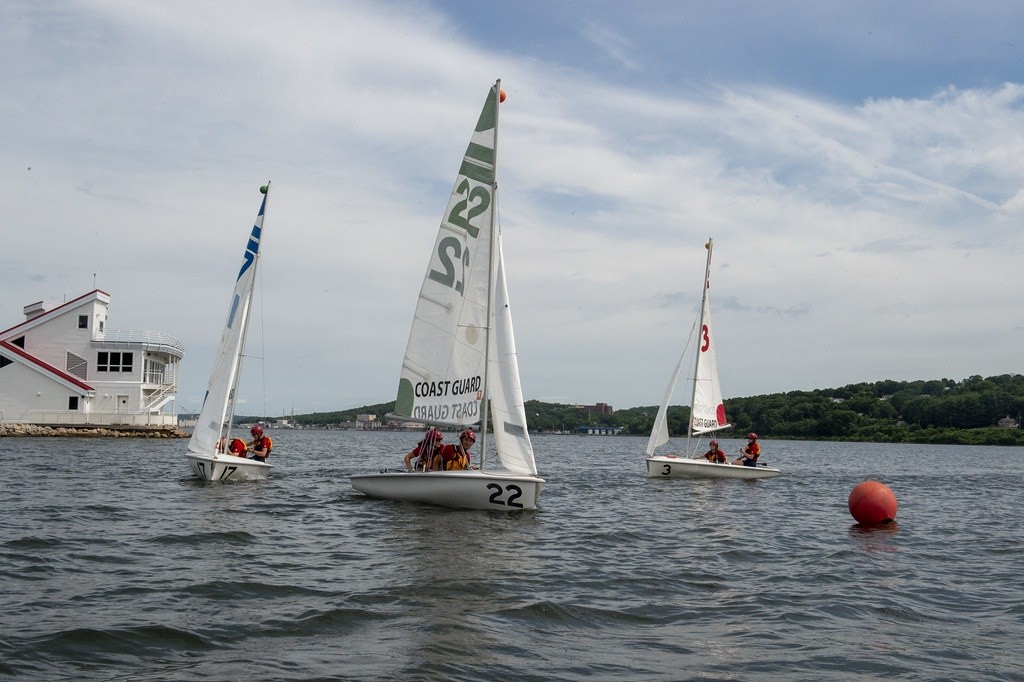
[460,430,476,443]
[709,441,718,447]
[251,426,263,437]
[426,430,443,440]
[748,433,757,440]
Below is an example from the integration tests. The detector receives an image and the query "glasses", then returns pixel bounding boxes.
[748,437,752,440]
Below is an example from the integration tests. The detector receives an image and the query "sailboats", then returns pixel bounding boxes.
[645,238,782,480]
[183,177,274,484]
[347,76,547,512]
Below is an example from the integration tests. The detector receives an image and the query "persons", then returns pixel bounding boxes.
[244,426,272,462]
[404,428,446,472]
[694,440,727,464]
[731,433,760,467]
[441,430,479,470]
[219,437,247,458]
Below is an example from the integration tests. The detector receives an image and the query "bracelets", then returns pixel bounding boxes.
[252,450,255,453]
